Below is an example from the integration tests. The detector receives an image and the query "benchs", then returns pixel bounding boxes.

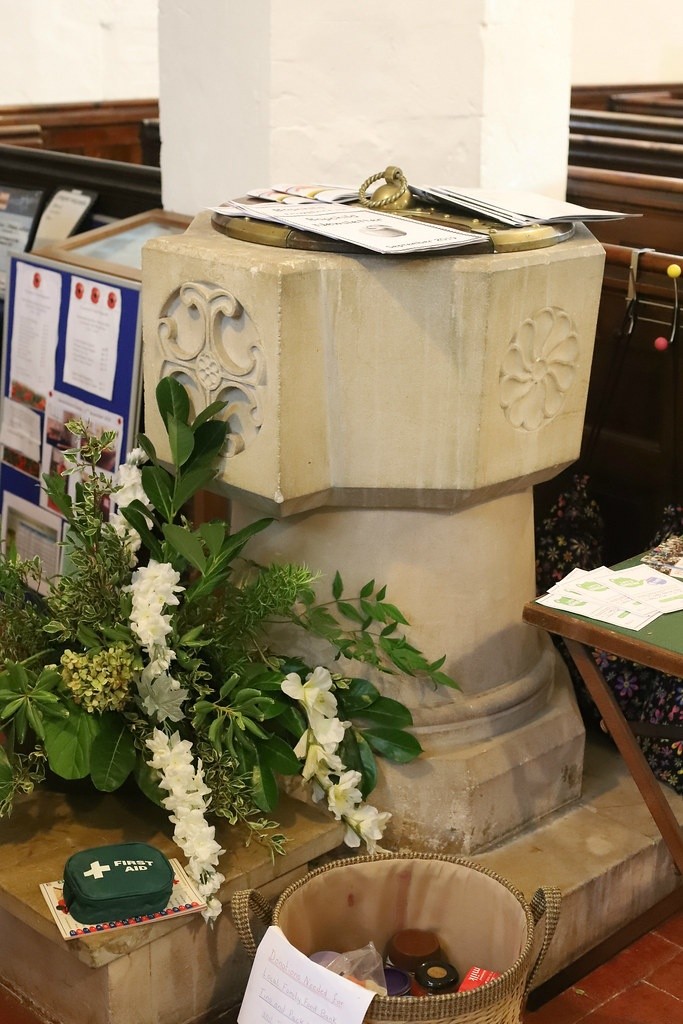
[0,77,683,567]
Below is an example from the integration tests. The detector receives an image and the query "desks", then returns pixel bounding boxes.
[521,529,683,879]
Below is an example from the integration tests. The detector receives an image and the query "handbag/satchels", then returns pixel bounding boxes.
[58,843,174,926]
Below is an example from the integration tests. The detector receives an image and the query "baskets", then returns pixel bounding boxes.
[230,851,563,1024]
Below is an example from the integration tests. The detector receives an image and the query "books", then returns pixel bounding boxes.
[38,857,208,941]
[640,534,683,579]
[228,183,644,254]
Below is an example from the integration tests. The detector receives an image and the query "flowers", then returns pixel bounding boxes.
[0,374,462,932]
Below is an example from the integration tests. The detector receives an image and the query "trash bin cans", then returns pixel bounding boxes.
[229,853,561,1024]
